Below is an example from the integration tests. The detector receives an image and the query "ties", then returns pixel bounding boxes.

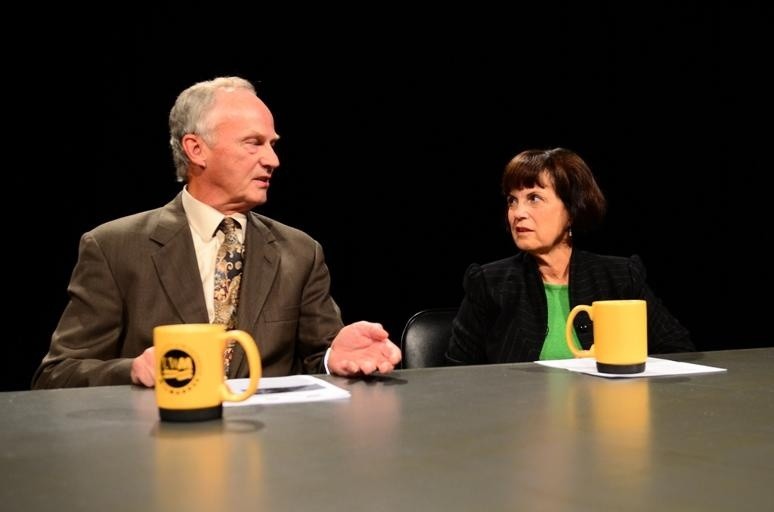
[211,217,246,379]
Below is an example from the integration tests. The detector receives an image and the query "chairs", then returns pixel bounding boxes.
[402,311,457,367]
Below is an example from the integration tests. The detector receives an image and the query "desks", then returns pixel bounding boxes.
[1,340,774,511]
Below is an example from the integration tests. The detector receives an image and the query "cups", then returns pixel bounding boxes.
[569,378,652,486]
[151,321,263,422]
[155,423,262,512]
[562,300,649,375]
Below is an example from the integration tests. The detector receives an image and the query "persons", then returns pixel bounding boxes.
[27,77,402,388]
[444,147,694,367]
